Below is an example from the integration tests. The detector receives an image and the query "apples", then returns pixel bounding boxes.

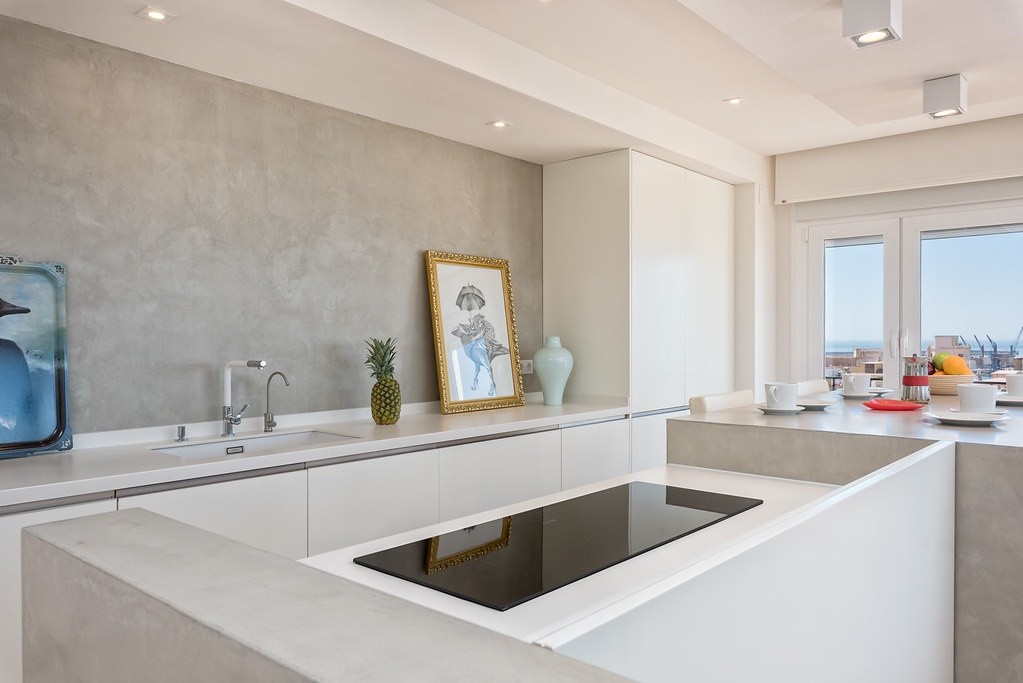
[927,362,936,374]
[931,352,951,370]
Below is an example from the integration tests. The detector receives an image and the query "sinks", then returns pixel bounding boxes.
[150,429,362,460]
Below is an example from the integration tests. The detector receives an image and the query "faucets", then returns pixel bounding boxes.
[222,360,267,436]
[263,370,290,433]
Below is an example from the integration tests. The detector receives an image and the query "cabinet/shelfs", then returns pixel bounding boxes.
[0,415,635,683]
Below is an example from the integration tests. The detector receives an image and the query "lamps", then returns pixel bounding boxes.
[922,74,968,120]
[842,0,902,50]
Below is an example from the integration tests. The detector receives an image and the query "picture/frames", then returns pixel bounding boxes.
[425,250,525,414]
[426,516,512,574]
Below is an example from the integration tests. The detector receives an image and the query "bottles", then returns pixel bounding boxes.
[533,336,574,405]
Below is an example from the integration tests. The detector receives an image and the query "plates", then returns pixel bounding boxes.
[862,398,925,410]
[756,405,805,415]
[996,391,1023,404]
[869,387,895,396]
[837,394,879,399]
[795,399,834,410]
[928,408,1010,426]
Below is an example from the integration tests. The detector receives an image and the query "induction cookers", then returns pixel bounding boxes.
[353,481,768,611]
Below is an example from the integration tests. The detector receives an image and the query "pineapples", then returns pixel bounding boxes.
[363,336,401,426]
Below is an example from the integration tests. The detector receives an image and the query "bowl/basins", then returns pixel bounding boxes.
[928,375,975,395]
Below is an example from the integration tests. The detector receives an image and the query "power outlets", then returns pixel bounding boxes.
[520,360,533,374]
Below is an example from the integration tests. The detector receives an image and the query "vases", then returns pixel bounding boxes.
[533,336,574,406]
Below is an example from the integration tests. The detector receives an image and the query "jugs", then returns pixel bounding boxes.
[900,356,931,404]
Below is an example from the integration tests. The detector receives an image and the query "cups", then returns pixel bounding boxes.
[1006,375,1023,396]
[765,382,798,408]
[957,383,998,412]
[842,373,870,393]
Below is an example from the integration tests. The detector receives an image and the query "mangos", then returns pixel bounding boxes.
[931,357,972,375]
